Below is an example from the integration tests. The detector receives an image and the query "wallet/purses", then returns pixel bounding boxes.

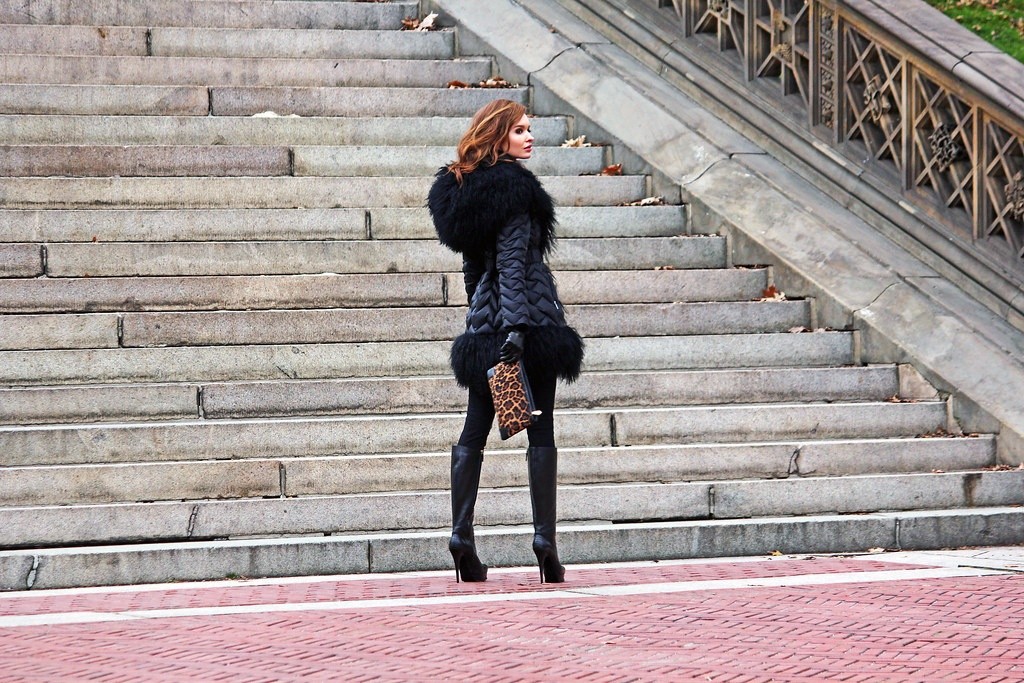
[487,358,542,441]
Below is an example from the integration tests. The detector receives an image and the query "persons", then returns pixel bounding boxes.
[425,99,584,584]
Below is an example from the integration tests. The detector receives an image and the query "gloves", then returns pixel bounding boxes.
[499,329,525,365]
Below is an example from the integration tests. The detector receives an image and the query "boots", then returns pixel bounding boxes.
[449,445,489,583]
[526,446,565,584]
